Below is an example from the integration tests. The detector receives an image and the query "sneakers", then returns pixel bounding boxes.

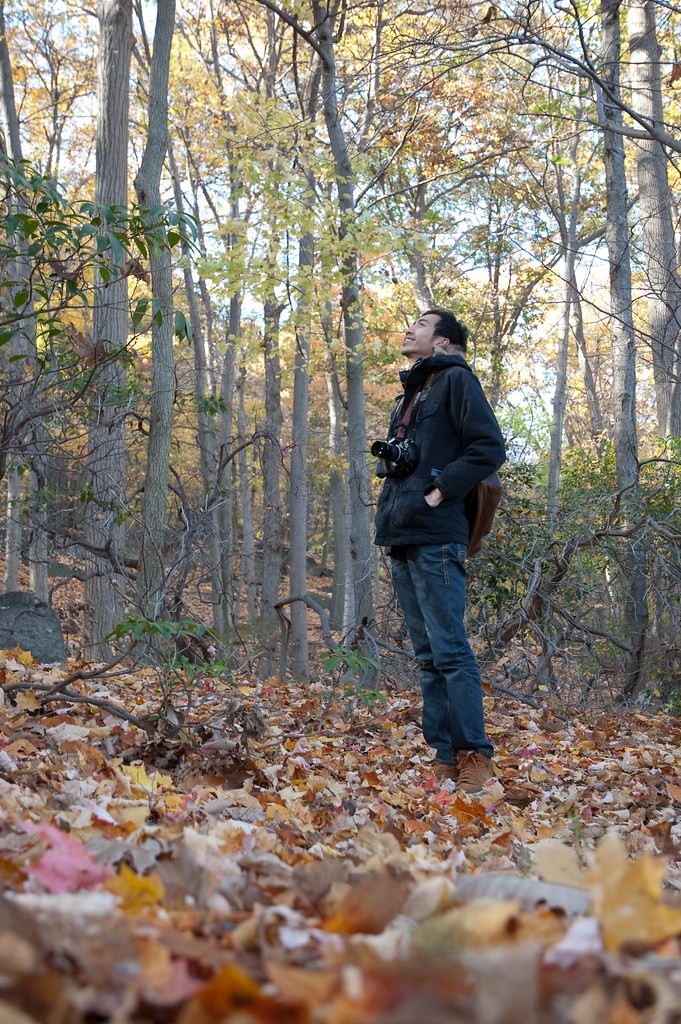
[452,751,492,794]
[431,764,459,780]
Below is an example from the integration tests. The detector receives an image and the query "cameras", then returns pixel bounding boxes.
[372,437,420,478]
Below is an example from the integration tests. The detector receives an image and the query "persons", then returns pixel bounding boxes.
[375,311,506,795]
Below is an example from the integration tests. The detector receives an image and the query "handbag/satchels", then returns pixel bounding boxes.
[467,472,502,557]
